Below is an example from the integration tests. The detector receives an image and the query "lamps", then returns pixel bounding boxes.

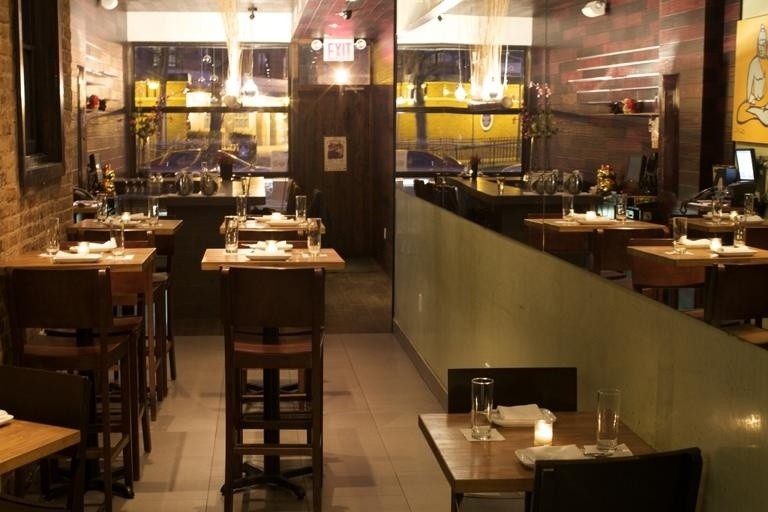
[96,0,120,11]
[445,1,517,108]
[194,46,225,105]
[307,35,368,65]
[576,0,614,19]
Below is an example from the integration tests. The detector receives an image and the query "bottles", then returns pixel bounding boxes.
[726,166,736,184]
[525,171,583,196]
[146,172,164,195]
[88,154,102,190]
[636,99,646,112]
[177,172,217,196]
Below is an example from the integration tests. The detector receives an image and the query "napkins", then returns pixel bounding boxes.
[527,444,582,466]
[496,404,540,421]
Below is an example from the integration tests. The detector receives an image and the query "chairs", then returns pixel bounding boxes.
[526,447,701,511]
[4,362,90,510]
[57,171,326,377]
[446,366,578,500]
[709,263,768,345]
[405,162,767,251]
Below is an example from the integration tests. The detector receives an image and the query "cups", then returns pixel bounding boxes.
[45,217,60,259]
[496,177,505,192]
[729,210,738,222]
[236,195,248,223]
[596,387,622,454]
[295,195,308,222]
[109,220,125,256]
[471,377,494,441]
[96,194,108,223]
[89,95,98,110]
[672,215,688,253]
[532,418,554,447]
[615,195,627,226]
[709,236,723,253]
[147,196,159,226]
[224,214,240,253]
[712,196,723,224]
[306,217,322,259]
[743,193,754,218]
[733,225,746,248]
[561,193,574,222]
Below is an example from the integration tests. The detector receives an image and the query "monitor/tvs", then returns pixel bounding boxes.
[736,148,758,181]
[625,155,646,184]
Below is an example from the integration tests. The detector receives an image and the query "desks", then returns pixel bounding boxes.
[3,246,159,419]
[203,246,346,399]
[413,408,656,510]
[1,417,80,474]
[628,243,767,311]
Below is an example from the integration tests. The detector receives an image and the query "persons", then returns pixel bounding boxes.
[737,25,768,126]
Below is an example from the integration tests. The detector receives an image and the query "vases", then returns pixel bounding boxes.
[468,162,479,180]
[524,133,553,176]
[219,163,233,185]
[134,135,160,170]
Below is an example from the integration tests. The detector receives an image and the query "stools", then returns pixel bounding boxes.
[216,264,325,506]
[2,265,136,511]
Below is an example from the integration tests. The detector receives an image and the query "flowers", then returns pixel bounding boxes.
[470,152,482,166]
[216,152,233,167]
[108,87,180,140]
[515,73,561,142]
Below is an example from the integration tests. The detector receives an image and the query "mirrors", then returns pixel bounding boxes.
[389,0,768,356]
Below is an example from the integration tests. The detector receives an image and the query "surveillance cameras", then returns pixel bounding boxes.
[343,11,352,20]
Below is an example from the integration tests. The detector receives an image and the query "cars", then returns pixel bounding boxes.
[140,151,271,171]
[394,148,463,171]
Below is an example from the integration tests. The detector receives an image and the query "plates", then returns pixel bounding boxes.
[572,213,612,225]
[52,244,113,263]
[702,214,729,220]
[682,244,710,249]
[253,217,300,227]
[717,246,757,257]
[485,408,558,430]
[0,414,15,427]
[245,242,295,261]
[740,218,763,225]
[106,213,147,227]
[512,448,536,470]
